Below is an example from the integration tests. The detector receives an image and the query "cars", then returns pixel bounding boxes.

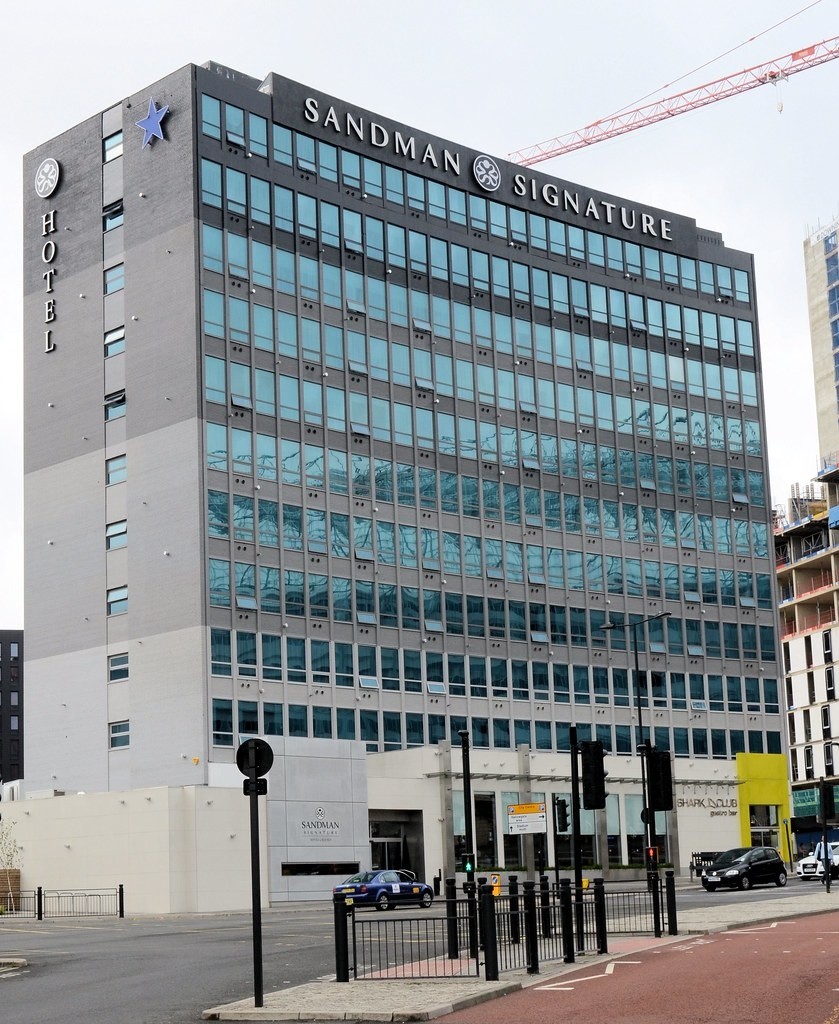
[701,846,787,892]
[796,843,839,882]
[332,870,434,911]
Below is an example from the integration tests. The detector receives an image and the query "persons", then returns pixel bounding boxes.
[798,843,807,860]
[809,842,816,852]
[814,833,833,886]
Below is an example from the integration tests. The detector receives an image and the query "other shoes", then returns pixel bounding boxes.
[830,882,832,885]
[820,880,824,885]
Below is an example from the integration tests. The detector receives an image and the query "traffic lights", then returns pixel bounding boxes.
[557,800,571,832]
[646,846,659,864]
[462,854,475,873]
[582,741,609,810]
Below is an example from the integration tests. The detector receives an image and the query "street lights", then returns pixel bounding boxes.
[598,611,672,850]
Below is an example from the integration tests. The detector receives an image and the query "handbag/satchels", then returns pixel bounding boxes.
[817,842,821,861]
